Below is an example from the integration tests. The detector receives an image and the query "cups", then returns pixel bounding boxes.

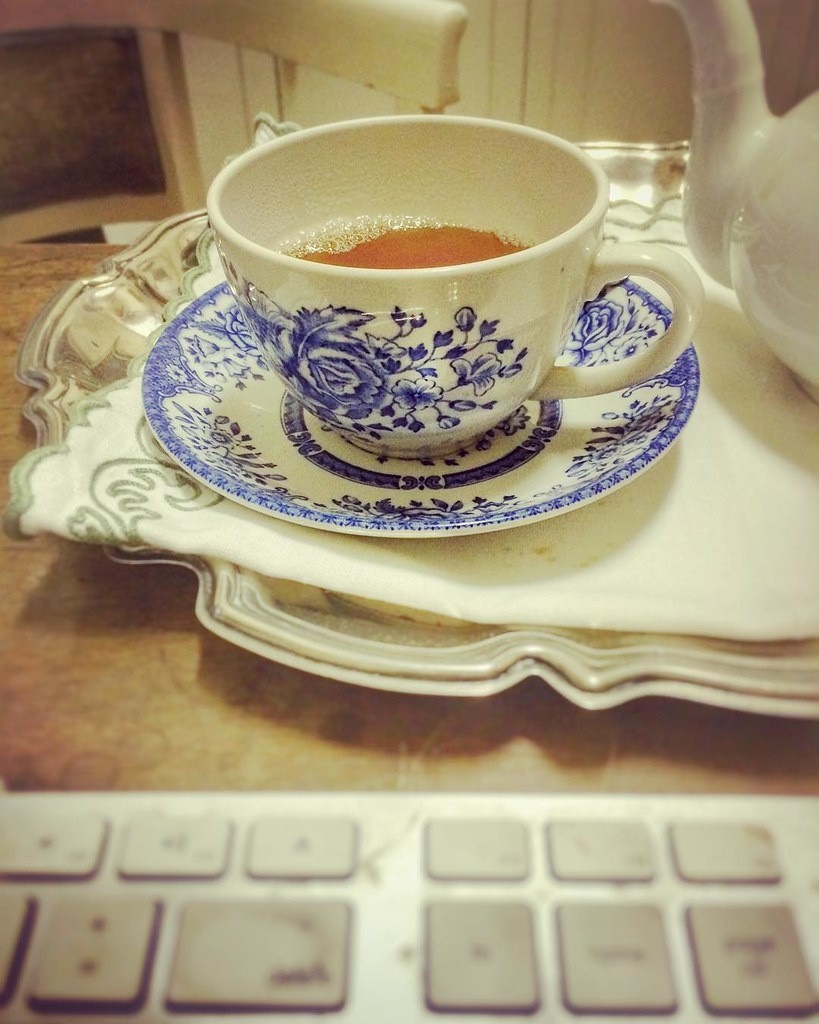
[205,113,702,459]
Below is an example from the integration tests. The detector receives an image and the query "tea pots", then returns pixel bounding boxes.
[638,0,818,404]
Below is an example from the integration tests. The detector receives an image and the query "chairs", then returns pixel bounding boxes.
[1,1,469,242]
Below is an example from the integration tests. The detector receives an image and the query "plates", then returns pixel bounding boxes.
[141,280,699,540]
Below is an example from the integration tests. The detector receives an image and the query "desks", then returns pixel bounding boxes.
[0,241,816,796]
